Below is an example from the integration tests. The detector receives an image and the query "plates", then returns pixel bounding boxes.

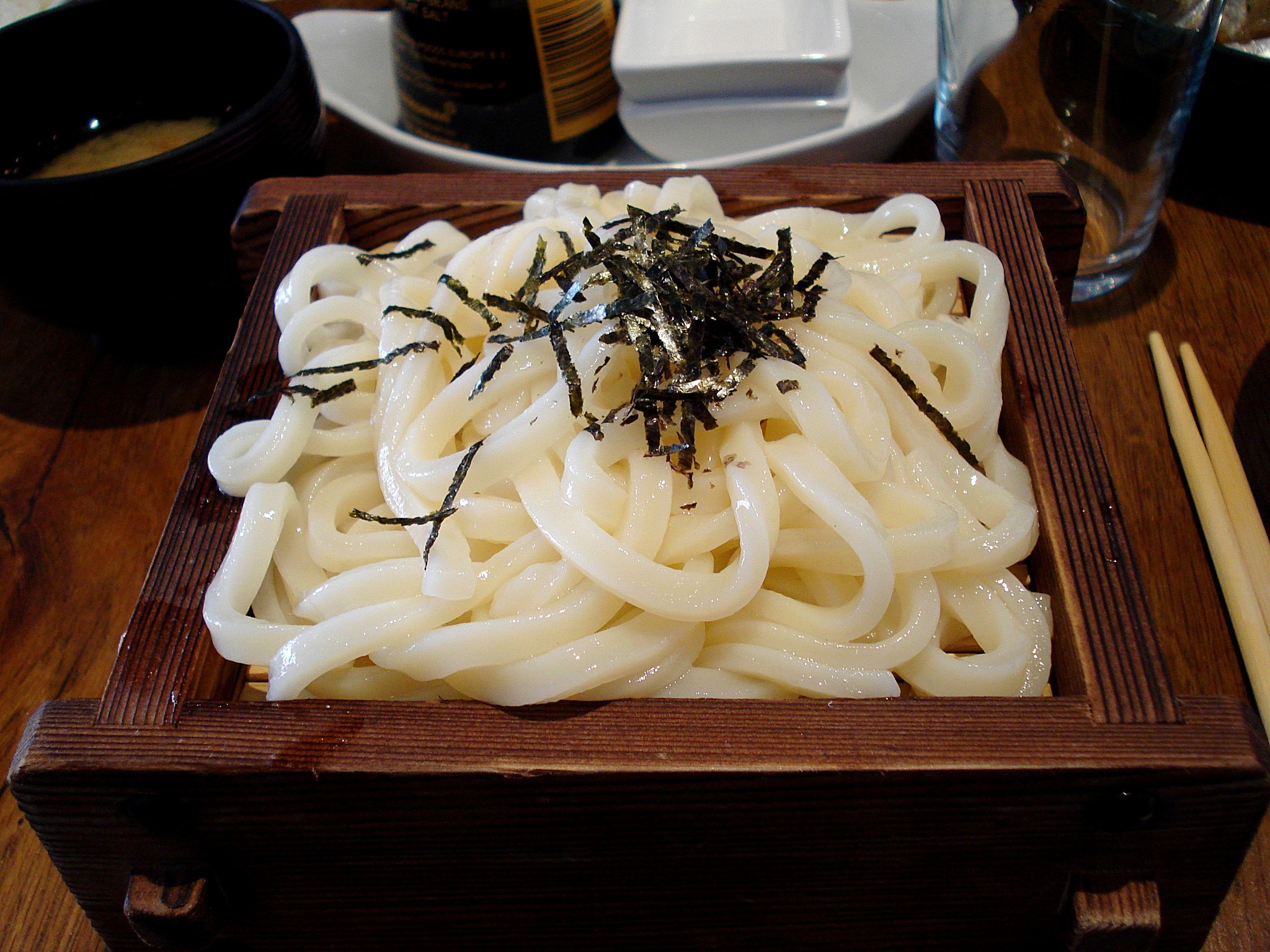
[288,0,1018,172]
[4,161,1270,952]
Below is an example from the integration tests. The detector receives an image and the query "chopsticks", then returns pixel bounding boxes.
[1149,330,1270,748]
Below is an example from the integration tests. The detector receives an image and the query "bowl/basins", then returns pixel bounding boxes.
[1109,0,1270,110]
[0,0,322,279]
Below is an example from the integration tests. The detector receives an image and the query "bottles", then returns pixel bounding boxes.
[389,0,626,164]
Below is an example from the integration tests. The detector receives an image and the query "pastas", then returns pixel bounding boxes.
[210,176,1052,704]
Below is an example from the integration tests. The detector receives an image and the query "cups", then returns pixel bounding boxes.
[936,0,1228,302]
